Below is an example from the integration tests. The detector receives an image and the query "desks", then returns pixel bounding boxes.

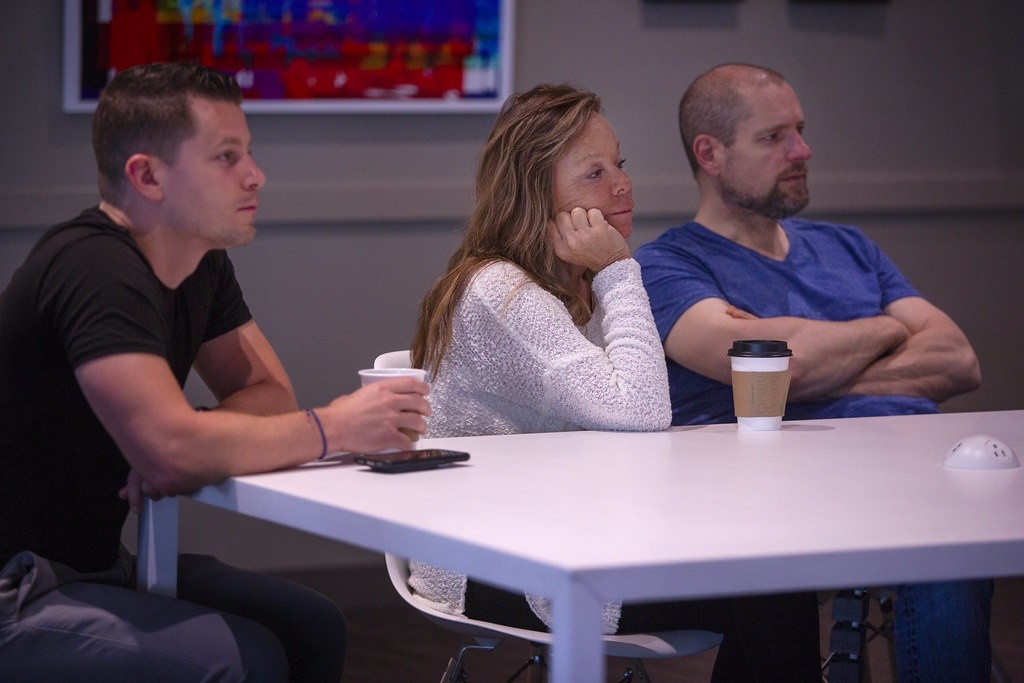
[143,402,1024,683]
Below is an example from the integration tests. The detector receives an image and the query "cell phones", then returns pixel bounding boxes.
[353,449,469,472]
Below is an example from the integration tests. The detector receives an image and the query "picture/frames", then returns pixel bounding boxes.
[60,0,521,118]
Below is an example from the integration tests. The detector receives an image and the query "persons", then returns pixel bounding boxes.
[405,82,820,683]
[631,63,998,683]
[0,62,434,683]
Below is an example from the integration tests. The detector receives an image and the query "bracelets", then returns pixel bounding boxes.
[306,405,328,460]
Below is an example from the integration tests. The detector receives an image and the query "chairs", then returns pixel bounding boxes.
[364,345,733,683]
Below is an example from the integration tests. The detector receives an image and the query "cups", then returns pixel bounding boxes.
[727,338,793,431]
[359,369,428,458]
